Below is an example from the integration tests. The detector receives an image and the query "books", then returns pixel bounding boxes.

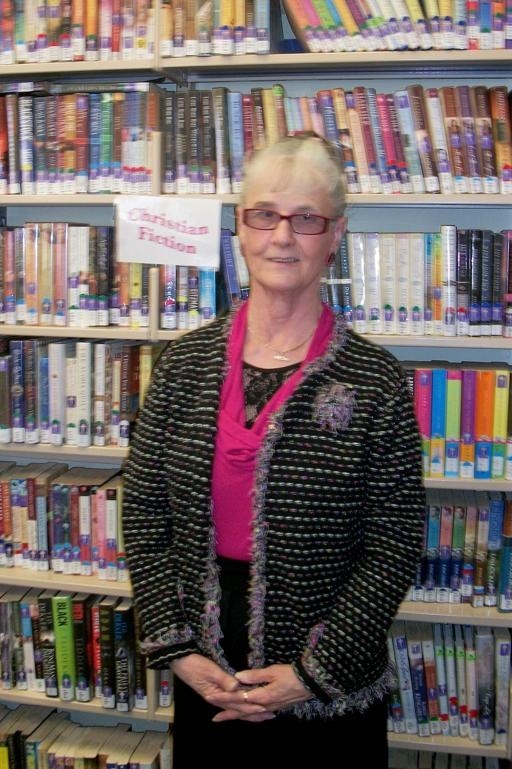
[0,335,162,449]
[1,222,251,332]
[0,584,174,713]
[0,0,512,65]
[382,619,512,769]
[0,702,174,769]
[400,359,512,482]
[404,488,512,612]
[0,79,512,195]
[318,224,512,338]
[0,460,131,583]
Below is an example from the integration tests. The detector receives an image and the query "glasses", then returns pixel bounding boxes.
[243,209,335,235]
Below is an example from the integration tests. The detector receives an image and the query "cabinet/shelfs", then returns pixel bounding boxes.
[0,2,512,768]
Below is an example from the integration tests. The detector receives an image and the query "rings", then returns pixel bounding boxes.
[243,690,248,702]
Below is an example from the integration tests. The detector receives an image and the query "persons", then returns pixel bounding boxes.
[122,139,428,769]
